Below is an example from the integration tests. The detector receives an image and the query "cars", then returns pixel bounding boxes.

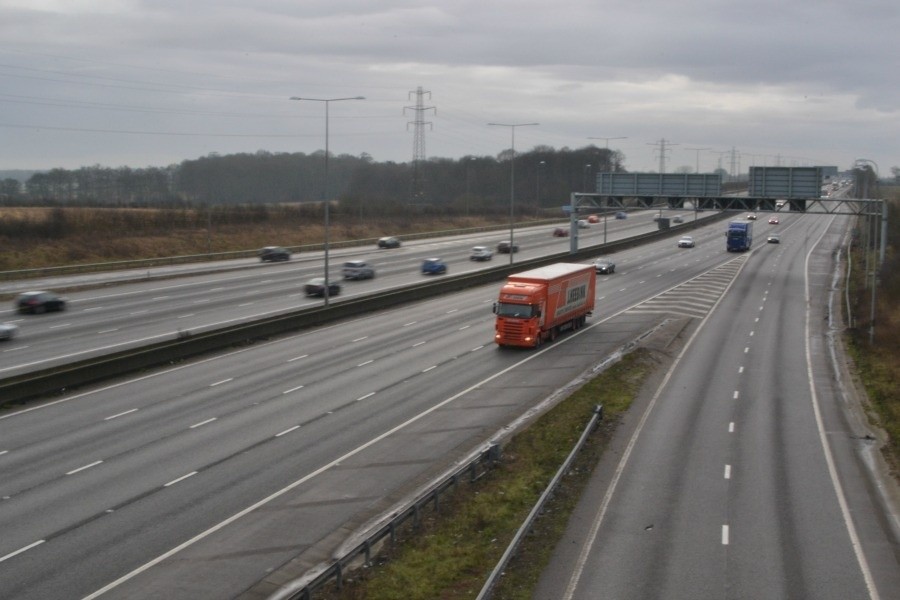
[421,258,447,275]
[615,211,627,220]
[342,260,375,280]
[0,322,19,341]
[776,200,784,208]
[768,216,779,225]
[14,290,68,315]
[588,215,601,223]
[594,258,615,275]
[678,235,696,248]
[653,213,663,222]
[303,276,341,297]
[766,233,780,244]
[672,214,684,223]
[496,241,518,254]
[469,246,494,261]
[577,219,590,229]
[822,178,854,197]
[256,245,290,262]
[553,228,569,237]
[747,213,756,220]
[378,237,401,249]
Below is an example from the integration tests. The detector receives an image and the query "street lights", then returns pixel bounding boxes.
[288,94,366,307]
[587,136,627,245]
[487,122,539,268]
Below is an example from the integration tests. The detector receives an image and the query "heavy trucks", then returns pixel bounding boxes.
[725,220,753,253]
[491,263,596,350]
